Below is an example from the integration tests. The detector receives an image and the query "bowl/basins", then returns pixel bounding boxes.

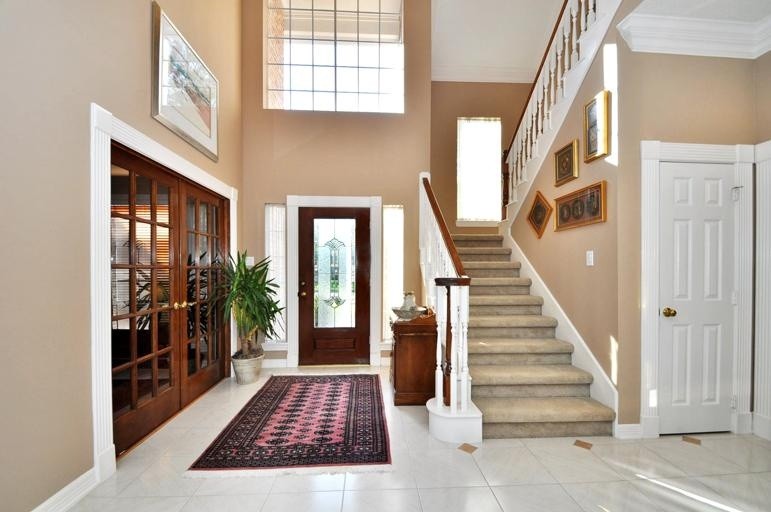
[390,307,427,320]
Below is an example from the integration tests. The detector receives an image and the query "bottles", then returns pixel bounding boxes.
[404,290,417,312]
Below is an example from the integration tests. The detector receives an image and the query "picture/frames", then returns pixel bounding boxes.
[149,0,222,164]
[526,88,612,240]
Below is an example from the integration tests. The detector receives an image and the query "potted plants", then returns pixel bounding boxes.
[129,249,205,376]
[205,246,288,386]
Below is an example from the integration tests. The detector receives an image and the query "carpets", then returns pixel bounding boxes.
[186,372,394,472]
